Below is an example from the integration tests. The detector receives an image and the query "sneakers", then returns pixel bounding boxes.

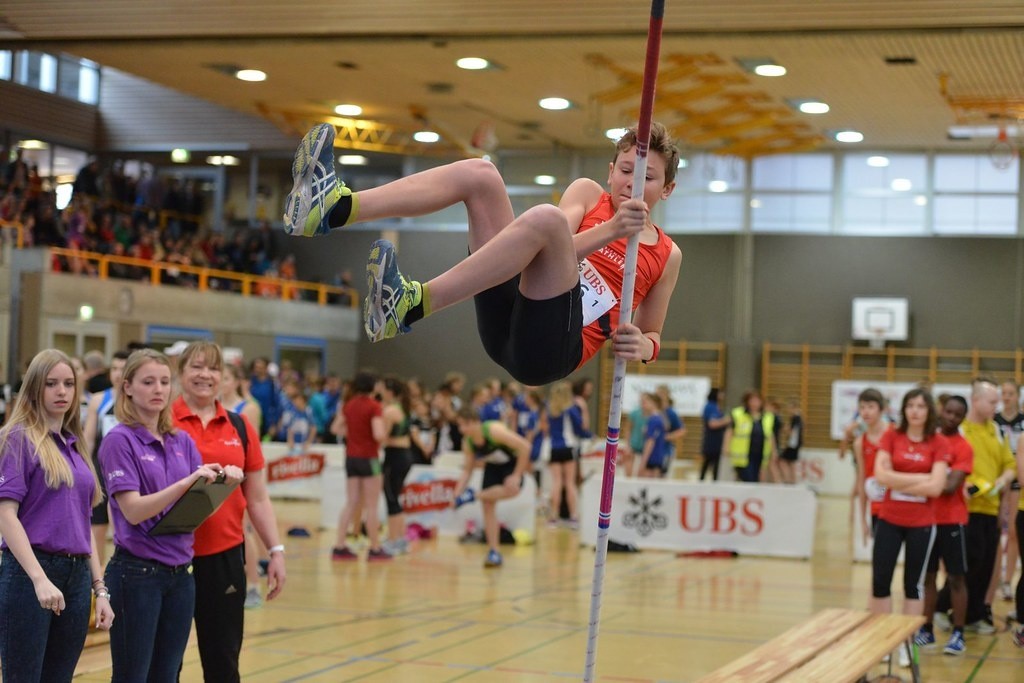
[282,122,352,237]
[332,547,357,562]
[368,548,392,561]
[944,632,967,655]
[363,239,416,344]
[914,630,935,649]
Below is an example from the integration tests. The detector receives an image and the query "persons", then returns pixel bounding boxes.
[0,344,803,606]
[0,348,115,683]
[283,123,683,386]
[839,379,1024,667]
[0,150,354,307]
[167,339,288,683]
[98,348,244,683]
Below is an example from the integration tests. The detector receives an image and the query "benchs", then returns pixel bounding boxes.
[702,605,928,683]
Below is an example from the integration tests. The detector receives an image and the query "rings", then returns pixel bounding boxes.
[229,471,234,477]
[110,624,112,627]
[51,605,59,610]
[46,605,50,608]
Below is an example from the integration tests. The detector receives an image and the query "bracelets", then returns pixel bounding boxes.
[96,593,111,601]
[267,545,284,558]
[92,581,105,589]
[642,337,658,366]
[94,587,108,595]
[92,579,105,584]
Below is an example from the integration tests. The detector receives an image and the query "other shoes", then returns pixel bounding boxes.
[964,620,998,635]
[450,489,475,508]
[1014,627,1024,647]
[900,641,913,668]
[486,550,501,566]
[933,610,951,631]
[1002,583,1012,602]
